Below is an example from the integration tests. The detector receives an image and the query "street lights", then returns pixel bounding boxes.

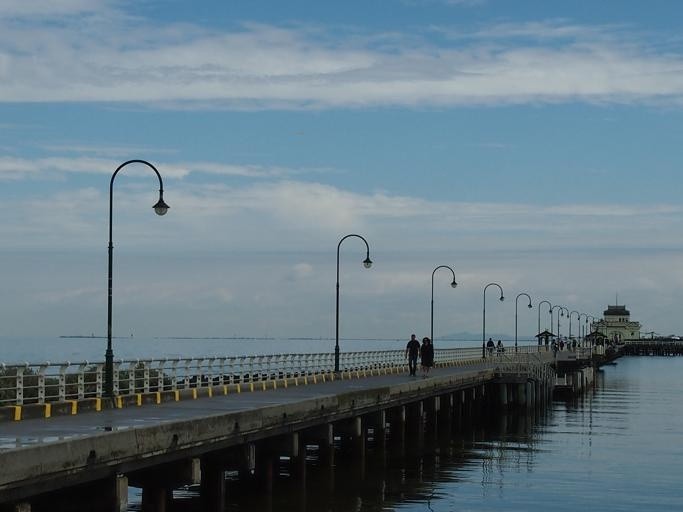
[103,160,169,395]
[430,265,457,344]
[482,284,600,358]
[335,234,372,372]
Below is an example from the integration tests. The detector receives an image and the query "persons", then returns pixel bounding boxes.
[420,337,434,377]
[551,339,576,358]
[496,340,504,357]
[405,334,421,377]
[487,338,495,356]
